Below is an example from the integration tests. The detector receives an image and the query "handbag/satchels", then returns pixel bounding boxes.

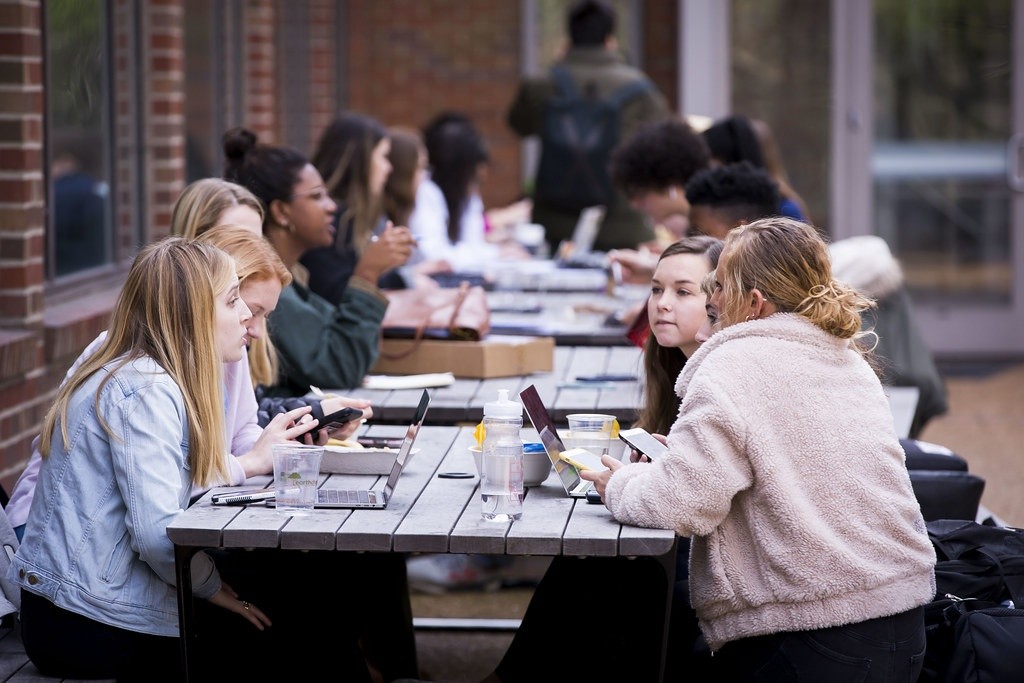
[923,519,1024,607]
[377,283,491,359]
[923,597,1024,683]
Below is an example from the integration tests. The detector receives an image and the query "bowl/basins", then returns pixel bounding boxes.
[320,445,422,476]
[560,435,627,463]
[469,445,553,487]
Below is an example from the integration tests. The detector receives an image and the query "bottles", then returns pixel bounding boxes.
[479,389,525,524]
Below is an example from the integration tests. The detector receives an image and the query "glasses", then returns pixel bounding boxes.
[279,185,329,204]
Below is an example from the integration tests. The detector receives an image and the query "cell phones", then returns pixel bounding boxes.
[619,427,669,464]
[294,408,363,444]
[211,487,276,504]
[559,448,610,472]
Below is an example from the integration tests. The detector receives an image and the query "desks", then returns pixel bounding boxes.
[372,285,655,343]
[165,424,681,683]
[297,343,649,428]
[465,251,626,286]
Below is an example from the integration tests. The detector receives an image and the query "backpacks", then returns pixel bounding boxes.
[540,65,653,215]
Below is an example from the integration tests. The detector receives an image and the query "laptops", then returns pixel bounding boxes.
[265,387,431,506]
[518,384,604,497]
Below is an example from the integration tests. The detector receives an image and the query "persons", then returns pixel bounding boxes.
[9,224,422,683]
[407,111,811,597]
[634,237,723,433]
[169,128,419,395]
[8,237,381,683]
[300,108,493,290]
[482,219,937,683]
[506,0,657,255]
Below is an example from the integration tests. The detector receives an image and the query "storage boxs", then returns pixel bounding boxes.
[373,337,554,378]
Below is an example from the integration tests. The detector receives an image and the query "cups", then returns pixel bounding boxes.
[566,412,616,457]
[271,444,325,516]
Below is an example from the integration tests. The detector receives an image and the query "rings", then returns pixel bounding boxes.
[243,601,249,610]
